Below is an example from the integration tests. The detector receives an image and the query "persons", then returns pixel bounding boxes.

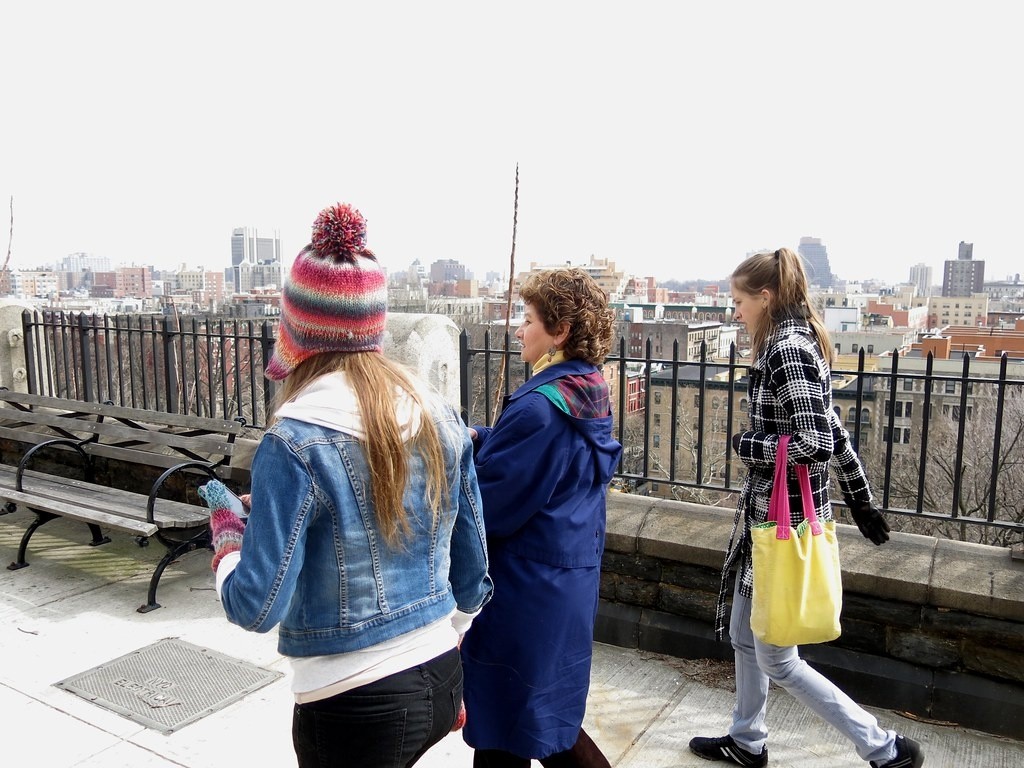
[205,203,494,768]
[689,246,927,768]
[459,268,611,768]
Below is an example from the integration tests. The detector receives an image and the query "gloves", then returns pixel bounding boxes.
[204,480,246,573]
[733,429,746,456]
[450,700,466,732]
[850,501,890,546]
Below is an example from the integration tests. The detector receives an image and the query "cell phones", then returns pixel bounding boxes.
[197,486,251,524]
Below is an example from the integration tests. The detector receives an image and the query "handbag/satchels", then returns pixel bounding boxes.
[749,435,843,646]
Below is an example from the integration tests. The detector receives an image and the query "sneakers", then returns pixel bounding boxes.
[870,735,925,768]
[689,734,769,768]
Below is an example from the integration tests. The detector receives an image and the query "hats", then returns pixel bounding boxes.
[262,202,388,380]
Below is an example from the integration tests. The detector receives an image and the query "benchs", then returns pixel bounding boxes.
[0,386,246,613]
